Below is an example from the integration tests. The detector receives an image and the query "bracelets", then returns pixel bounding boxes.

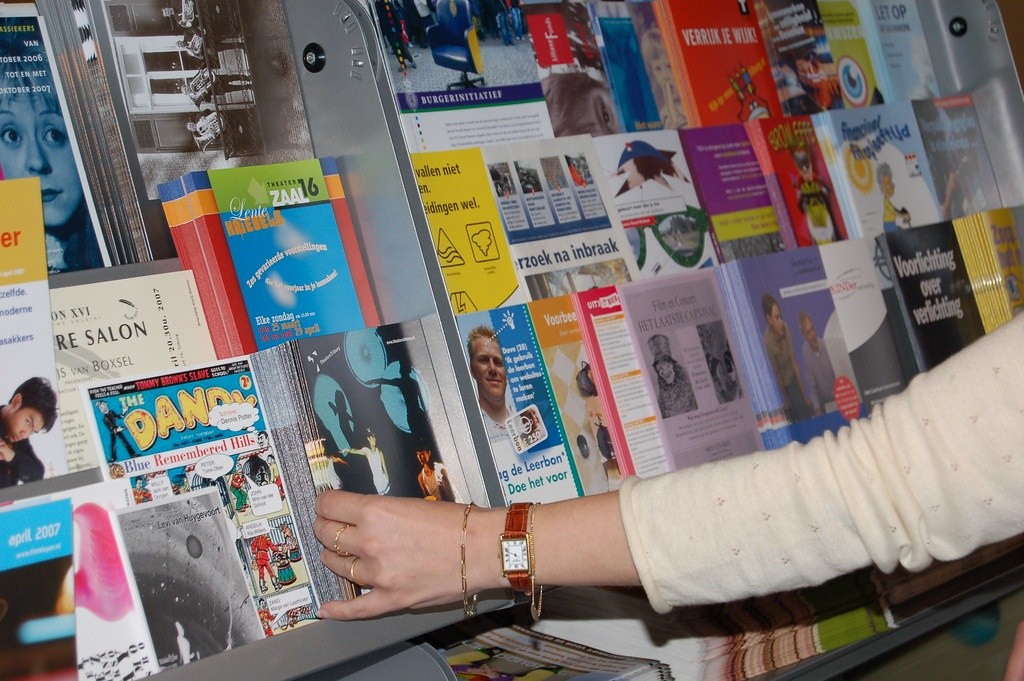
[460,501,478,615]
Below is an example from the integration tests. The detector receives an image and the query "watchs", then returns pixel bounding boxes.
[497,502,542,620]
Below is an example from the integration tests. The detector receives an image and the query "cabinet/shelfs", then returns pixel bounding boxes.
[0,0,1024,681]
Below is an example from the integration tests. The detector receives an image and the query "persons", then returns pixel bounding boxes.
[416,439,455,502]
[799,312,842,416]
[340,428,396,496]
[0,17,104,274]
[780,49,845,118]
[762,294,814,421]
[540,71,621,138]
[0,378,58,489]
[317,310,1023,620]
[466,322,519,441]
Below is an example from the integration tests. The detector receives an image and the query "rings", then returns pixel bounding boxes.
[351,558,364,585]
[334,524,354,557]
